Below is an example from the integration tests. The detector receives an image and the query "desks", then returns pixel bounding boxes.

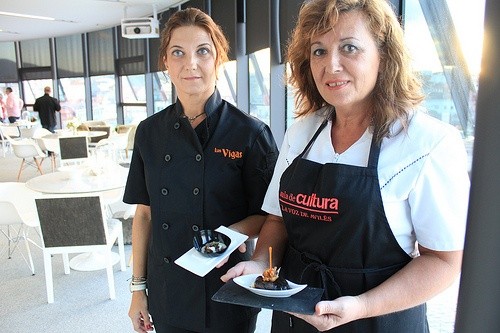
[43,130,108,138]
[26,165,130,273]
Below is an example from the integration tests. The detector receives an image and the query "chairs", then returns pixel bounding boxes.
[0,120,137,304]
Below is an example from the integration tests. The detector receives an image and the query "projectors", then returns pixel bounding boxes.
[121,17,161,39]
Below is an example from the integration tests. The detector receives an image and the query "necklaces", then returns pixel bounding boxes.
[187,111,206,124]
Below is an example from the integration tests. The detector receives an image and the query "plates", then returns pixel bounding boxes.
[232,274,308,298]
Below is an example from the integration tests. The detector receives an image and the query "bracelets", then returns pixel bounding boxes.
[126,275,146,292]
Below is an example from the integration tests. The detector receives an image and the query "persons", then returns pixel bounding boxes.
[122,9,279,333]
[33,87,61,133]
[219,0,471,333]
[4,87,23,123]
[0,93,6,141]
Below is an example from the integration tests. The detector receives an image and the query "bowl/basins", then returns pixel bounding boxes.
[193,230,231,257]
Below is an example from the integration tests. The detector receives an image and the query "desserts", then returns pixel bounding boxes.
[253,266,291,291]
